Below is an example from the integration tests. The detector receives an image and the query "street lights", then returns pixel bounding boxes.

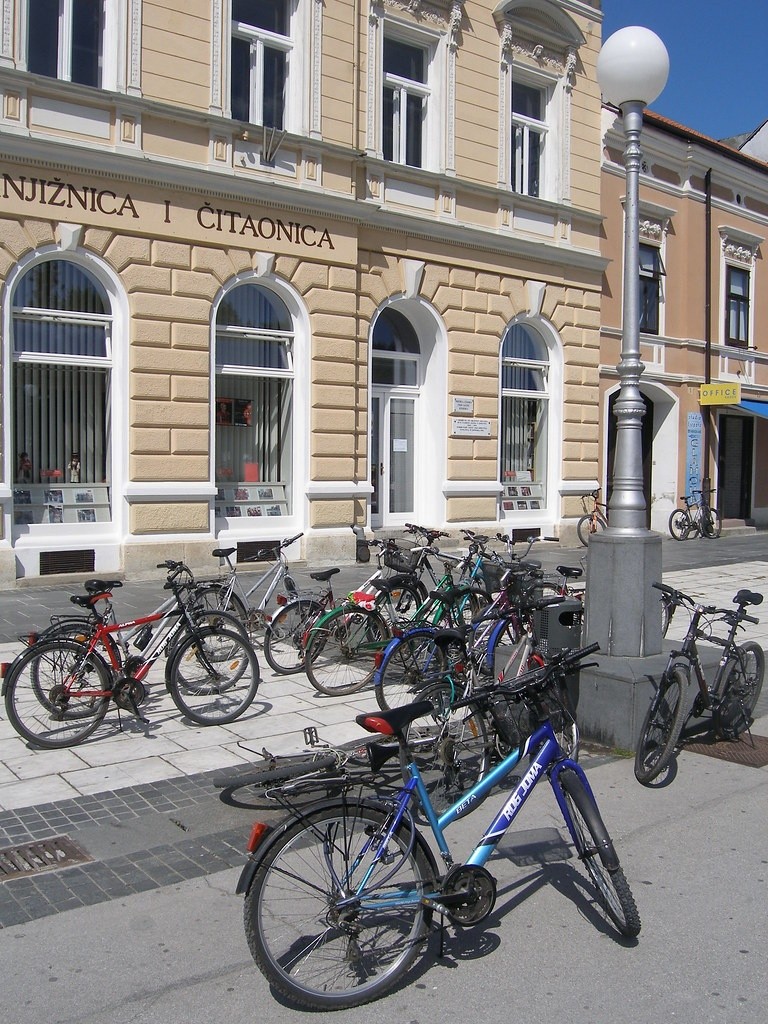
[593,26,676,662]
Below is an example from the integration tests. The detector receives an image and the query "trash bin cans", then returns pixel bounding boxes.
[531,595,582,709]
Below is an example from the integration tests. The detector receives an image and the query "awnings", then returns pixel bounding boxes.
[724,400,768,421]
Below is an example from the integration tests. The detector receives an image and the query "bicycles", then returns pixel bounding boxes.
[233,643,641,1011]
[0,523,671,823]
[577,486,611,546]
[635,577,766,781]
[668,489,723,543]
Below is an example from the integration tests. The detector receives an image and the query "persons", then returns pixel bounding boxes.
[217,403,231,423]
[67,452,81,483]
[14,452,32,484]
